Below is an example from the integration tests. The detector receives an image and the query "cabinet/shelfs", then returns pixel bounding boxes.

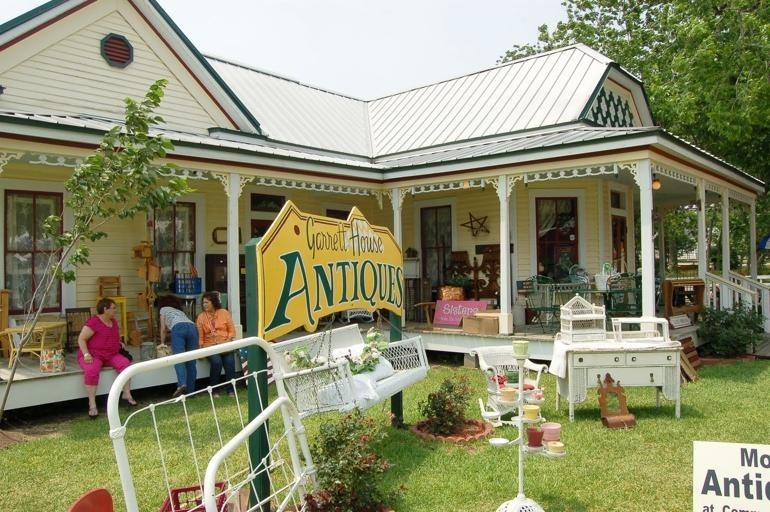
[405,277,423,324]
[549,335,685,423]
[152,293,205,343]
[559,292,608,345]
[97,295,127,346]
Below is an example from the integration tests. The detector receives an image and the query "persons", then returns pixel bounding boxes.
[196,292,237,399]
[76,297,139,417]
[157,295,197,398]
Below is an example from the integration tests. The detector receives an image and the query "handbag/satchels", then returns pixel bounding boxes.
[39,347,65,373]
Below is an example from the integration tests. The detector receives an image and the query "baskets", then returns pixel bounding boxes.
[608,273,635,291]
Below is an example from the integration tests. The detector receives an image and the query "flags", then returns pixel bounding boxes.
[516,280,536,294]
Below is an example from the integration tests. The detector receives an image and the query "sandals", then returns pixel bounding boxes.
[87,407,97,418]
[122,395,138,406]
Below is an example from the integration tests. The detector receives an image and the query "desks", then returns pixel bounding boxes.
[0,319,69,372]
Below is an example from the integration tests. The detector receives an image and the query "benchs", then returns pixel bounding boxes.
[271,323,432,419]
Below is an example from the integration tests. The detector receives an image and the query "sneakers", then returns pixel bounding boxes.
[174,383,187,398]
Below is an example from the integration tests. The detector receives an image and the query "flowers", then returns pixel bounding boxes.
[282,327,390,378]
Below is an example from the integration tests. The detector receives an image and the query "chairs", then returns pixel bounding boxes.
[341,309,375,325]
[65,306,93,352]
[468,343,549,429]
[604,274,642,332]
[554,271,590,328]
[524,274,561,335]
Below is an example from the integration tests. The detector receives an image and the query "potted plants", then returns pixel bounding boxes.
[403,246,421,278]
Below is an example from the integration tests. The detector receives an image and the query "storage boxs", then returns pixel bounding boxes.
[460,308,499,337]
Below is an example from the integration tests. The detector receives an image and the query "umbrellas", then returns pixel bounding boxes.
[758,235,770,249]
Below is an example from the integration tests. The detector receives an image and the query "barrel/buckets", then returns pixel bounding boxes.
[140,342,157,362]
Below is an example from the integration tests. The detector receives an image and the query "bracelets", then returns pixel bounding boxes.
[83,353,91,357]
